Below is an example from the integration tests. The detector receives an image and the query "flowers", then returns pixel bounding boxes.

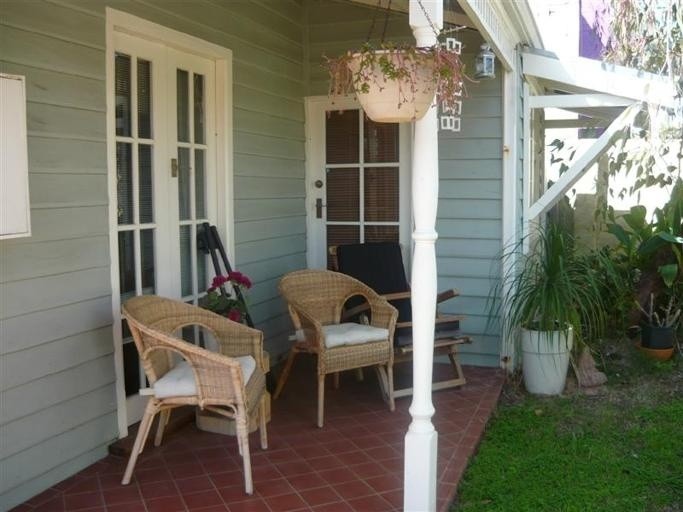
[200,270,251,325]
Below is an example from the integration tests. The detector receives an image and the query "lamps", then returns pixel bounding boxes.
[473,44,497,80]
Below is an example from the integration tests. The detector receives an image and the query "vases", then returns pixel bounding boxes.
[639,323,676,361]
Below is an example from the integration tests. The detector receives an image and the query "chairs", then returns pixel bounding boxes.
[120,294,268,496]
[271,270,399,426]
[327,240,473,403]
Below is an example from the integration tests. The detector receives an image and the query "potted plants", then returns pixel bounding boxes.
[478,208,617,398]
[319,40,468,127]
